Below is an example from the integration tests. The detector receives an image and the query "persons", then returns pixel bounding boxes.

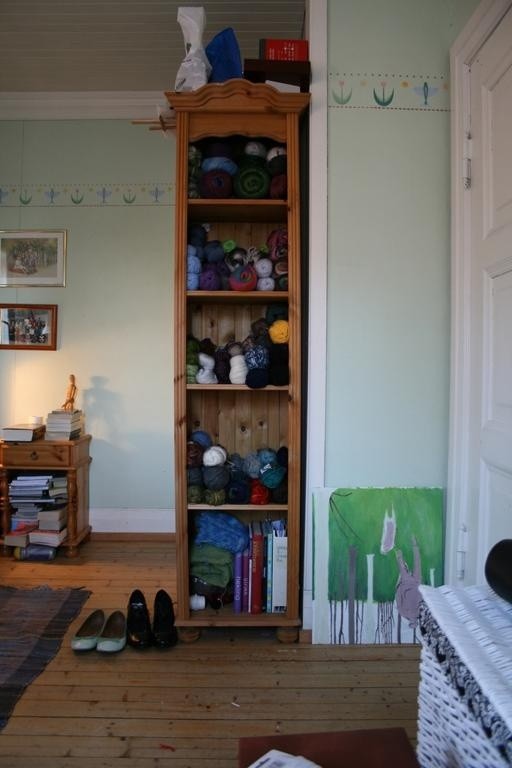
[2,316,45,343]
[9,240,53,275]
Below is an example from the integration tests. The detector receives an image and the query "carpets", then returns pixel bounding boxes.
[1,583,94,729]
[237,726,420,768]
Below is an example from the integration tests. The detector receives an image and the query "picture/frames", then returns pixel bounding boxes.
[0,229,68,351]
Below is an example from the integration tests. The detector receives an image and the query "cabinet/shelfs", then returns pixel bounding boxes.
[1,440,91,557]
[165,79,311,645]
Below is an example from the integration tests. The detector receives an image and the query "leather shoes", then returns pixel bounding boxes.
[126,589,152,651]
[152,589,179,649]
[70,608,107,652]
[95,609,127,654]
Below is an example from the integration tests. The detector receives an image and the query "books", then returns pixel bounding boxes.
[4,474,68,549]
[1,423,46,442]
[44,409,82,440]
[234,518,286,615]
